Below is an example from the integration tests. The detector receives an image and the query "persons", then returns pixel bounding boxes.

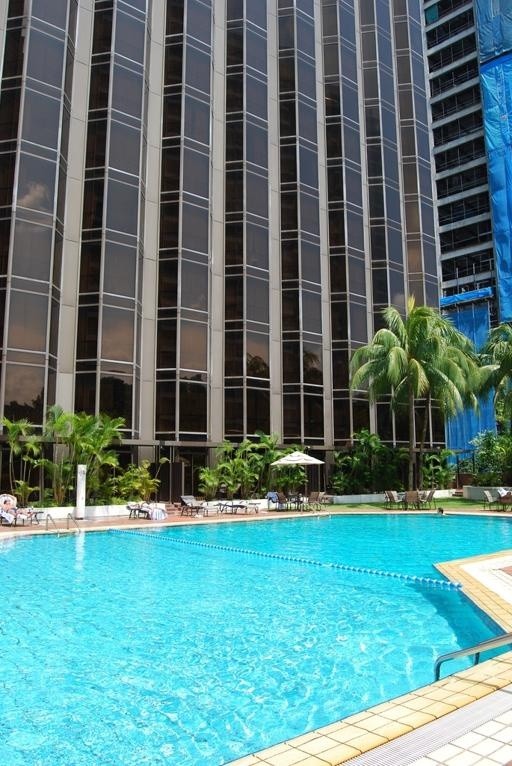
[276,489,287,504]
[1,499,33,518]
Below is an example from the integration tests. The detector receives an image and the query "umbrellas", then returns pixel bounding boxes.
[270,451,325,500]
